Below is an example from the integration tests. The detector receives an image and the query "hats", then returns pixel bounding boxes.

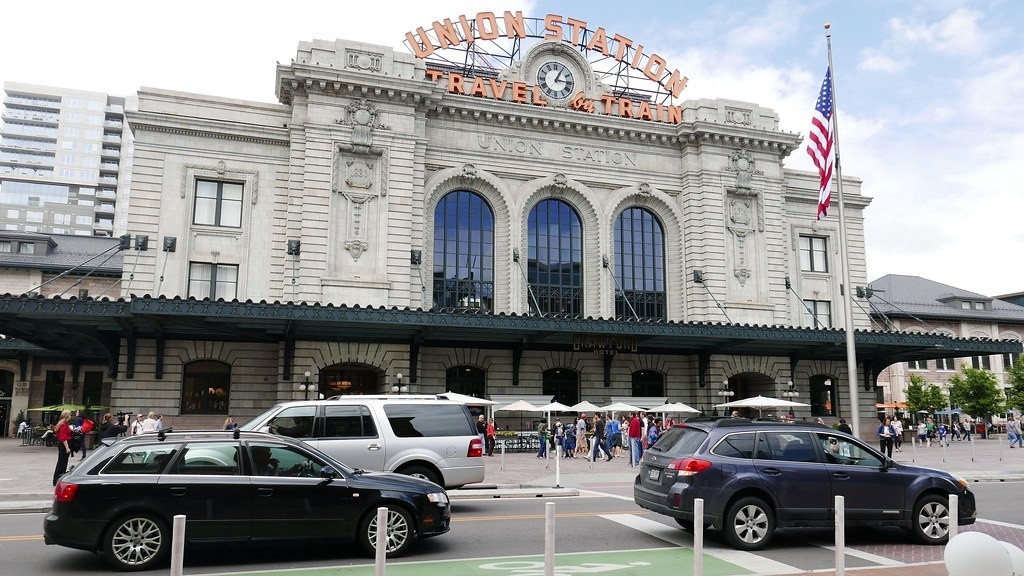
[581,414,587,418]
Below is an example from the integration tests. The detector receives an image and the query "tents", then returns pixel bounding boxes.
[23,403,113,424]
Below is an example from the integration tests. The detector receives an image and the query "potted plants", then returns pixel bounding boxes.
[80,397,98,450]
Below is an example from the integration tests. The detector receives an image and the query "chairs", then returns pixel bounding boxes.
[505,434,539,453]
[784,440,800,450]
[769,436,780,450]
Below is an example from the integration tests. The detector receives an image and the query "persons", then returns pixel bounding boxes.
[52,409,72,486]
[17,411,238,461]
[476,411,676,469]
[729,410,1024,458]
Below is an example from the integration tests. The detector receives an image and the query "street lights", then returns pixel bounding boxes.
[297,371,316,402]
[391,373,407,395]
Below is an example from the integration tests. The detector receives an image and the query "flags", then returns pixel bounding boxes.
[806,65,836,222]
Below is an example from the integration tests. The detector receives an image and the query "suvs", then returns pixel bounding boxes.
[43,424,451,571]
[184,392,486,495]
[633,416,978,551]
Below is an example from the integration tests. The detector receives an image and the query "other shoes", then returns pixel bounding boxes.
[898,447,901,452]
[606,456,613,461]
[587,459,596,462]
[583,456,589,459]
[896,449,898,452]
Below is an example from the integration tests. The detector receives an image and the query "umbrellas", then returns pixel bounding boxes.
[497,400,702,451]
[712,394,812,419]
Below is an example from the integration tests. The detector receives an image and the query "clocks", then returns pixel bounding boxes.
[536,60,574,100]
